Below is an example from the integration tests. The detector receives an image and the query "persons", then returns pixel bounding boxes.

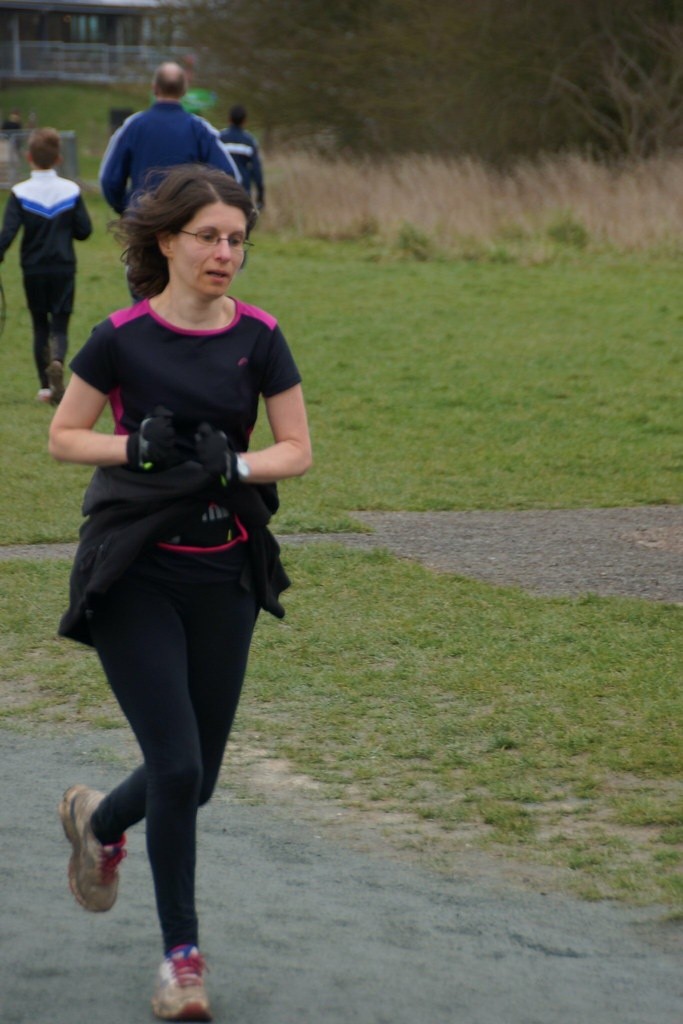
[99,62,242,303]
[217,105,266,269]
[48,165,313,1020]
[0,126,94,400]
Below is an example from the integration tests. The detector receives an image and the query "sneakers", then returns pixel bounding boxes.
[59,783,126,912]
[151,944,212,1020]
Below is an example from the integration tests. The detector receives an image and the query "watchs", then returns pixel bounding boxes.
[236,454,249,477]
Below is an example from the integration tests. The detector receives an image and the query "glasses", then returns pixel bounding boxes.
[179,230,255,250]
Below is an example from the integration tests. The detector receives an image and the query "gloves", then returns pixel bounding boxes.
[128,405,238,488]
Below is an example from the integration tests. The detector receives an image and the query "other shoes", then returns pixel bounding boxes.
[49,361,65,405]
[38,389,52,397]
